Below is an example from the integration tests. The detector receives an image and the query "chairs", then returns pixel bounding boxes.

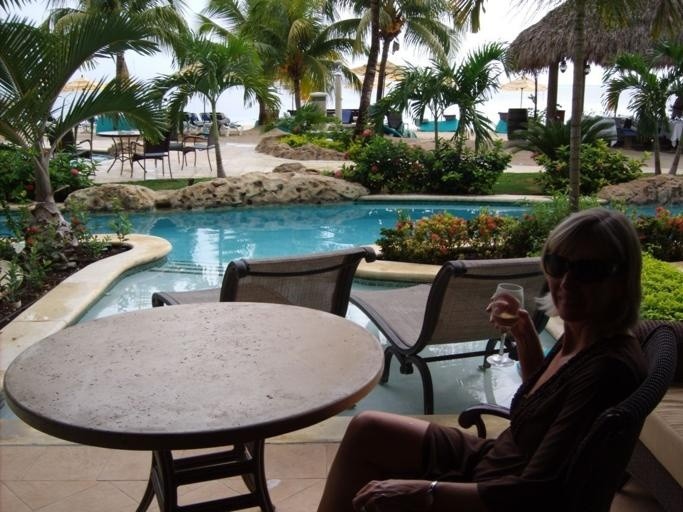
[128,117,229,184]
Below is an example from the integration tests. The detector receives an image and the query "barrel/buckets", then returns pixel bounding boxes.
[506,108,528,142]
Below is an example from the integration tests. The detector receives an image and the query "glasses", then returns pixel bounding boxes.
[543,254,613,282]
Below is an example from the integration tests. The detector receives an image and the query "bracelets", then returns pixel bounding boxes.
[425,479,437,510]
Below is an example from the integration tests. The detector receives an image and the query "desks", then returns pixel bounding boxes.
[2,301,385,511]
[96,129,146,178]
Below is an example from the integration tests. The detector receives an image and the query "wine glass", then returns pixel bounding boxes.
[483,282,526,368]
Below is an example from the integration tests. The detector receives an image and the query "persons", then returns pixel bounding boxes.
[316,206,645,511]
